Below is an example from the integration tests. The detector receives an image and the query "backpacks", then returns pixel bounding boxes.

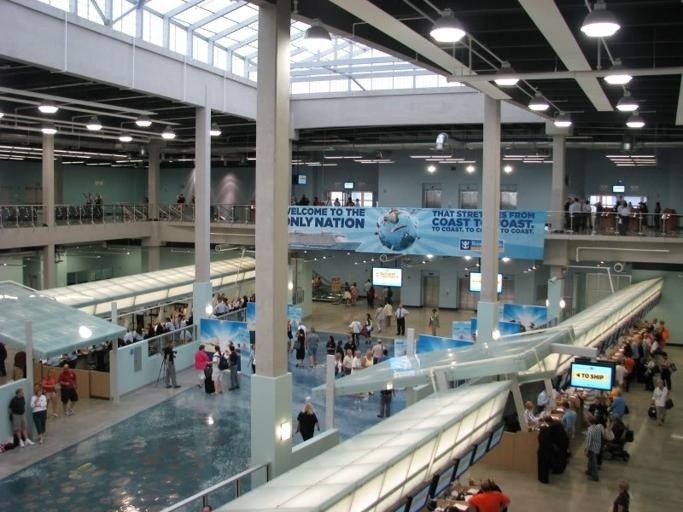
[217,355,229,370]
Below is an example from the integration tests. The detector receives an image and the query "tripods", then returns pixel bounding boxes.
[156,358,168,385]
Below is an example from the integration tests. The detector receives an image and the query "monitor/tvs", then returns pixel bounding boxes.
[570,362,612,391]
[371,267,403,288]
[575,358,615,386]
[469,272,503,294]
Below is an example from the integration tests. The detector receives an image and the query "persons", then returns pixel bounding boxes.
[286,272,443,441]
[467,478,511,512]
[522,317,677,482]
[291,193,361,207]
[612,483,630,512]
[563,195,661,236]
[176,193,195,221]
[80,192,106,218]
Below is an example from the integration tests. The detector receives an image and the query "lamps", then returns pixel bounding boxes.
[580,2,622,39]
[300,2,335,44]
[29,100,223,143]
[428,2,468,43]
[493,54,646,129]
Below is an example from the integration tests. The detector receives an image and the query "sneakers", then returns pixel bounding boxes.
[52,408,76,417]
[19,439,35,448]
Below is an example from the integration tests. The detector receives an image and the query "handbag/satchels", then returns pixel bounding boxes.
[293,341,301,350]
[648,406,657,419]
[665,399,674,409]
